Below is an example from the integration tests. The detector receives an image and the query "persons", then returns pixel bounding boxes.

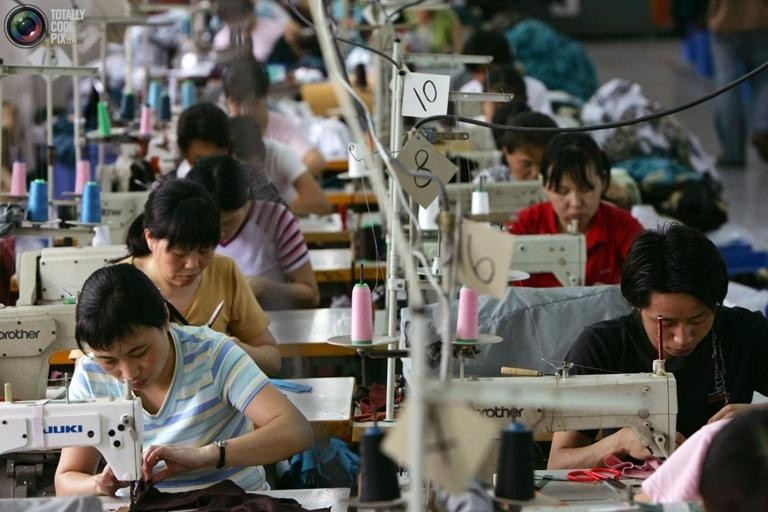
[446,28,557,123]
[545,218,766,474]
[690,412,767,512]
[703,0,766,168]
[50,263,317,500]
[499,129,654,289]
[207,56,329,182]
[105,175,283,382]
[457,61,534,132]
[173,99,295,210]
[466,107,563,182]
[179,152,322,312]
[171,113,334,218]
[204,1,438,89]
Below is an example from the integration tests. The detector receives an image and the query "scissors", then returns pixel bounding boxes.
[566,467,627,491]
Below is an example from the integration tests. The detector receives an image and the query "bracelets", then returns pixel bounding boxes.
[213,439,227,469]
